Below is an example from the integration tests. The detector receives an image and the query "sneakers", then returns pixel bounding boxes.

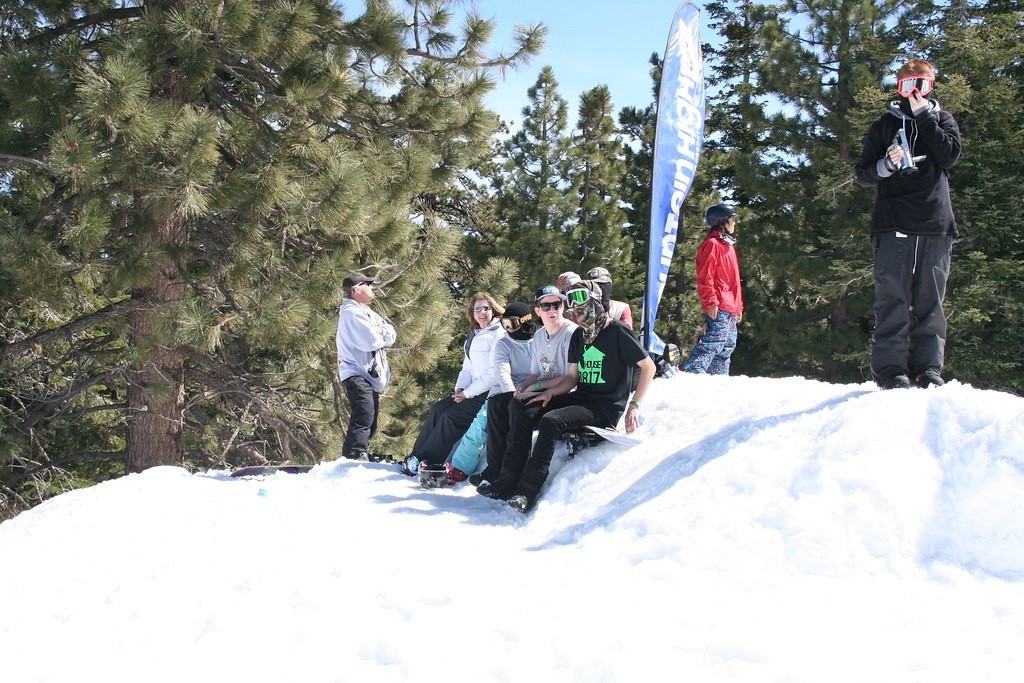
[875,366,910,389]
[506,487,533,514]
[909,368,945,388]
[477,485,514,501]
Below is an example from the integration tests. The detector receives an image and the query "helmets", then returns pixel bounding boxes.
[705,203,737,227]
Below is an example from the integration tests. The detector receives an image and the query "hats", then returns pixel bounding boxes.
[585,267,613,284]
[502,302,533,324]
[342,272,374,292]
[556,272,581,293]
[535,286,567,301]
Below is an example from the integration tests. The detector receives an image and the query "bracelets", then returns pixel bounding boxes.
[536,380,540,391]
[629,400,639,408]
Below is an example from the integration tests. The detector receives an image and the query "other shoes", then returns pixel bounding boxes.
[469,473,486,486]
[401,456,426,477]
[449,467,468,481]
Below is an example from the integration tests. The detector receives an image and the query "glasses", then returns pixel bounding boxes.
[897,75,934,97]
[537,301,562,312]
[473,306,491,312]
[500,316,522,330]
[353,281,370,291]
[566,288,591,308]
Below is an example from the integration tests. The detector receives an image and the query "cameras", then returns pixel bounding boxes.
[892,129,927,178]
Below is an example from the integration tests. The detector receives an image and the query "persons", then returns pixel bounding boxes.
[335,272,396,463]
[680,203,743,377]
[855,58,961,391]
[398,265,654,514]
